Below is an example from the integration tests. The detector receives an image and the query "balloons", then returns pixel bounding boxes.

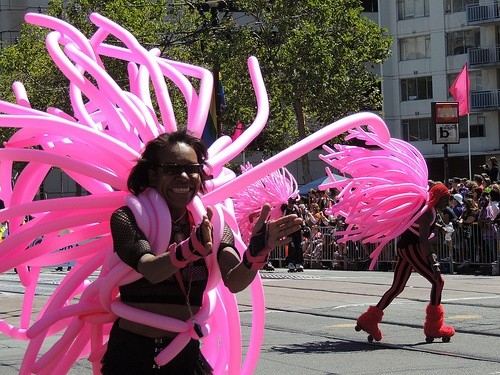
[0,11,393,375]
[317,125,436,270]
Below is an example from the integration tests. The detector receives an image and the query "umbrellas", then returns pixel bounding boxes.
[298,175,348,194]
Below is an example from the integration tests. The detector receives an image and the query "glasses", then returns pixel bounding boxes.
[162,163,203,173]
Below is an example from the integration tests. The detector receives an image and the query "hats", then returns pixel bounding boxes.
[454,194,463,205]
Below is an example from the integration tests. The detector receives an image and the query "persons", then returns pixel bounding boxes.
[261,158,500,273]
[100,128,301,375]
[55,244,80,271]
[355,185,455,342]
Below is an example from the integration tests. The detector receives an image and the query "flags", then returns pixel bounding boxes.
[232,124,242,142]
[449,65,470,116]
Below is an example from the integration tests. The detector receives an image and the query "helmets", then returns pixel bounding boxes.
[288,197,296,203]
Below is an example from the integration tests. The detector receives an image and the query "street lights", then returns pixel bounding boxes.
[207,0,221,139]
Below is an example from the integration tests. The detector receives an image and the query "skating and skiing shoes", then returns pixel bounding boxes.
[268,262,274,271]
[296,264,304,272]
[424,305,455,342]
[355,306,384,343]
[288,263,295,273]
